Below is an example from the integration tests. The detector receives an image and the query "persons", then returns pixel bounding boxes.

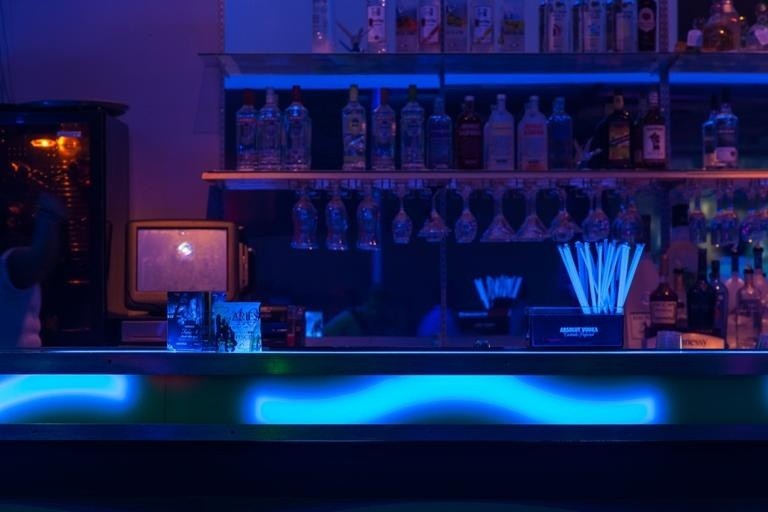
[670,143,703,243]
[326,284,408,338]
[508,277,529,340]
[0,192,66,348]
[414,285,462,336]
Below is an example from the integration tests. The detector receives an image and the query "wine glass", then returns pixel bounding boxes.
[289,181,649,251]
[678,183,768,244]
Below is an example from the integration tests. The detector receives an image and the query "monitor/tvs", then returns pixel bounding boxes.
[128,219,240,305]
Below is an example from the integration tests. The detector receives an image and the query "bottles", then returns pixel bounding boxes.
[649,240,766,352]
[364,3,768,53]
[305,0,335,55]
[717,86,740,172]
[698,87,716,169]
[234,81,667,174]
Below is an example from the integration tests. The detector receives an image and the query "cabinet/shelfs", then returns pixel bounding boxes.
[195,51,768,190]
[0,106,131,344]
